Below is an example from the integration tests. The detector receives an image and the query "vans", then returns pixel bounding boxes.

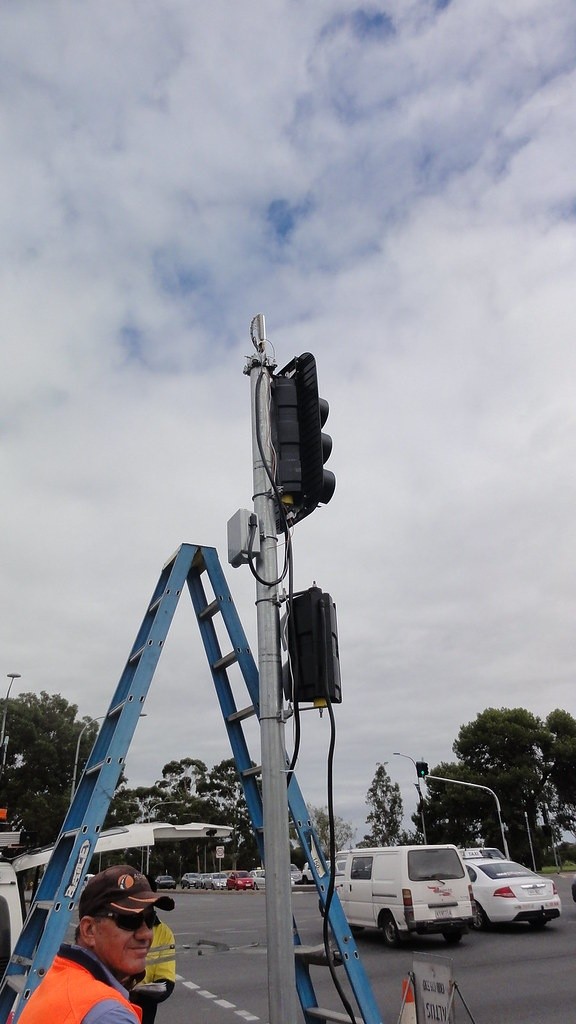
[333,844,475,949]
[302,861,331,884]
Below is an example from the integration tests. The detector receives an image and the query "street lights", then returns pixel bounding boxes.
[393,753,427,845]
[0,673,21,745]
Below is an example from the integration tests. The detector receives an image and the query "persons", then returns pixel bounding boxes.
[17,866,175,1024]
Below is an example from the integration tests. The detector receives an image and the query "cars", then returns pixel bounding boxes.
[249,870,266,885]
[227,871,255,890]
[194,874,210,889]
[204,873,229,890]
[155,876,176,890]
[463,858,562,934]
[459,848,505,862]
[290,864,302,884]
[180,873,199,889]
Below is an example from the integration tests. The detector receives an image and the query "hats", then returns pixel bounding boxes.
[79,865,175,921]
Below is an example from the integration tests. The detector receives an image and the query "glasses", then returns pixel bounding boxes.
[90,910,157,931]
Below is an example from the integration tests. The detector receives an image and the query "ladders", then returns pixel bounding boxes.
[0,541,386,1023]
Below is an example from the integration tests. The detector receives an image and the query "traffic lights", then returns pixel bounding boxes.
[416,762,426,777]
